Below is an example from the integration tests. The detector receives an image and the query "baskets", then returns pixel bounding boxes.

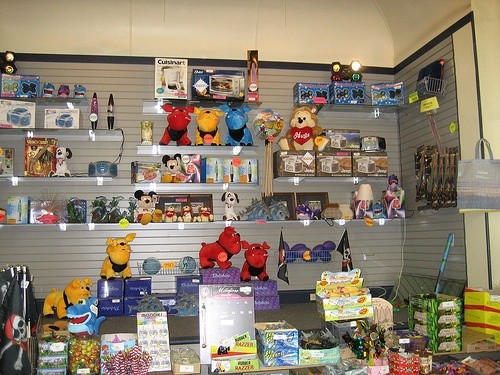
[137,262,199,275]
[284,250,333,264]
[416,76,448,100]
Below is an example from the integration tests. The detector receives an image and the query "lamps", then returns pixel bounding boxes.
[330,60,363,82]
[0,51,17,74]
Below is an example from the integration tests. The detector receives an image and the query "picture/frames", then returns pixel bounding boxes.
[261,192,296,220]
[155,194,213,210]
[296,192,329,206]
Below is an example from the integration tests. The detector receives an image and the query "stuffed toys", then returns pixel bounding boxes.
[220,104,253,146]
[199,226,241,269]
[42,278,93,319]
[159,104,194,146]
[52,147,72,177]
[277,106,330,152]
[374,201,386,218]
[193,107,225,146]
[296,203,320,220]
[135,190,162,225]
[100,233,136,280]
[164,206,177,222]
[182,206,192,223]
[222,192,240,221]
[240,240,270,281]
[199,206,211,222]
[66,298,106,335]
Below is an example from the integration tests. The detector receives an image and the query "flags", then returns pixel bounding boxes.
[0,267,38,375]
[277,231,289,285]
[335,229,353,272]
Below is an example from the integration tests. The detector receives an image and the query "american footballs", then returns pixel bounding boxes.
[283,240,336,263]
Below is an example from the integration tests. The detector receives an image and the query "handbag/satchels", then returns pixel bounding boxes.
[457,138,500,213]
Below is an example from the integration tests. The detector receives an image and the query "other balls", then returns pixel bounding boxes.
[143,256,161,275]
[178,256,196,274]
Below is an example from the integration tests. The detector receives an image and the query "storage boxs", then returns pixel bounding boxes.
[0,50,500,375]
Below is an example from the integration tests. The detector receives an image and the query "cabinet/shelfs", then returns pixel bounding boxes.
[0,97,406,233]
